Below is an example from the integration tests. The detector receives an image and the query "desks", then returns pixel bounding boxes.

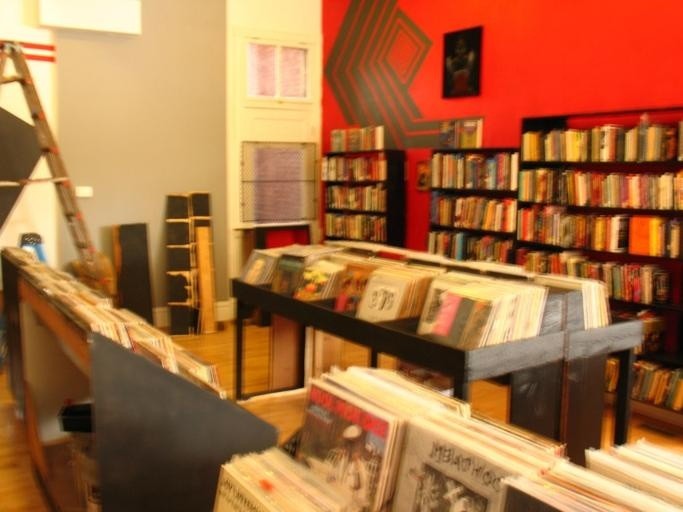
[230,279,644,442]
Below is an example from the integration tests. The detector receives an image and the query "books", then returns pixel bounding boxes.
[4,246,226,400]
[419,117,683,411]
[240,247,450,353]
[212,366,683,512]
[321,126,386,242]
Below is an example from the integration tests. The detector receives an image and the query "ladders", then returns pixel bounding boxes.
[0,42,97,276]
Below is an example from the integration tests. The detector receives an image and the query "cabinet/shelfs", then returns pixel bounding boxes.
[511,105,683,439]
[428,146,520,268]
[319,149,405,245]
[0,244,226,510]
